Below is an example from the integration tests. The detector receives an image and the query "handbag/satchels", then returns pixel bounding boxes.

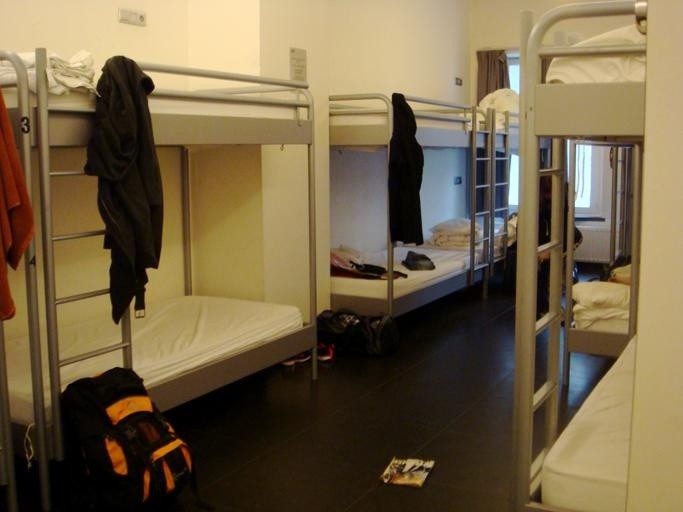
[317,307,401,359]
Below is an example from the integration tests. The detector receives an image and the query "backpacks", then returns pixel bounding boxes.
[401,250,436,271]
[58,366,196,512]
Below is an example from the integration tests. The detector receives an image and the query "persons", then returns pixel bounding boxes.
[540,176,583,327]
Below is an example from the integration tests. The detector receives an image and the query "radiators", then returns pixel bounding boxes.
[573,223,610,266]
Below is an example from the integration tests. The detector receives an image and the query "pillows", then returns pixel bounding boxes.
[427,219,485,234]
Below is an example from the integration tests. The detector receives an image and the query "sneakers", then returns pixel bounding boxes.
[279,342,335,366]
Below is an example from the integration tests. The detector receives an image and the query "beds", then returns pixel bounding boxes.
[23,43,321,470]
[610,143,644,282]
[423,103,552,289]
[325,93,490,342]
[0,48,49,510]
[509,0,652,512]
